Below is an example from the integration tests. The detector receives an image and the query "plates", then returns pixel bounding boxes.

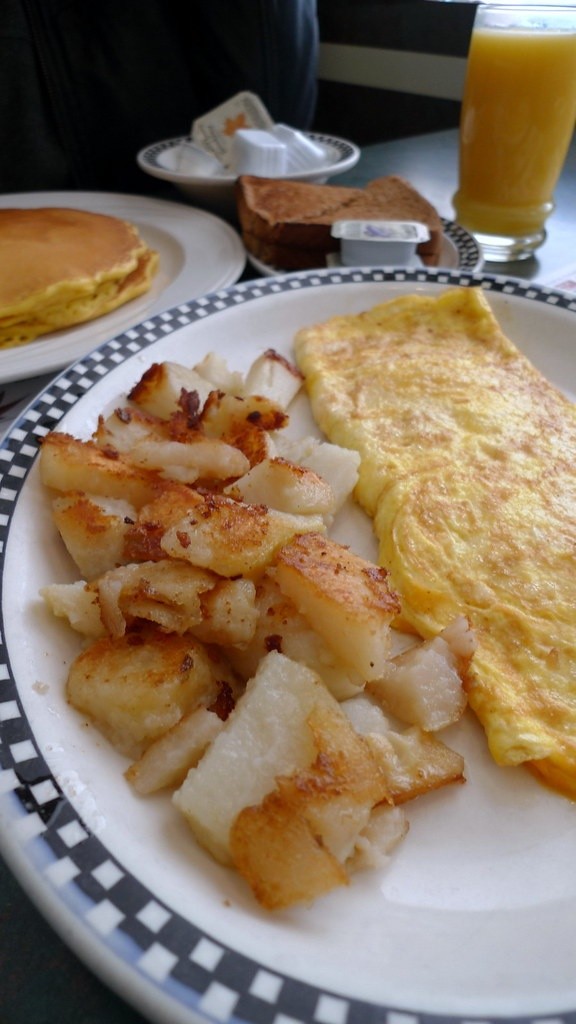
[0,265,576,1023]
[0,192,245,387]
[137,132,359,201]
[247,214,484,272]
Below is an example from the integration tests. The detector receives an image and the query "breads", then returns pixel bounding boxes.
[233,172,441,269]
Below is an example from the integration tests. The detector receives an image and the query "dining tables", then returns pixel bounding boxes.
[0,127,576,1024]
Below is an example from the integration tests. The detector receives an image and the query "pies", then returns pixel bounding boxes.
[291,283,576,805]
[0,207,160,346]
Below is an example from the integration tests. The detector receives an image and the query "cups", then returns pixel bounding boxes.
[451,5,576,263]
[331,218,429,265]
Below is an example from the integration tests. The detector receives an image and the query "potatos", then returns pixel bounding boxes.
[33,349,467,913]
